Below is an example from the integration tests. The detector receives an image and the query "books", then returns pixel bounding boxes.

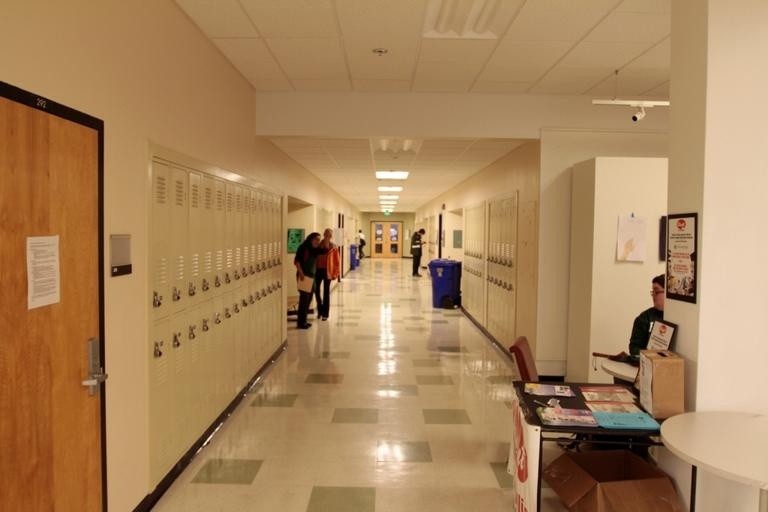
[522,381,661,431]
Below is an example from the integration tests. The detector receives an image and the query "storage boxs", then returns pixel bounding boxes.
[541,450,678,511]
[639,349,686,419]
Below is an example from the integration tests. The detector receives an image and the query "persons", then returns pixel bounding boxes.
[410,229,427,277]
[628,274,664,356]
[293,232,321,328]
[315,228,340,321]
[359,230,366,259]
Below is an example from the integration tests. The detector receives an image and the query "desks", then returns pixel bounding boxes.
[512,381,697,511]
[601,355,639,384]
[659,410,768,512]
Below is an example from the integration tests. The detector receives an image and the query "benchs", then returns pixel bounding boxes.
[288,295,314,315]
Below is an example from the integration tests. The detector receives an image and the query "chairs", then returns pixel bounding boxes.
[510,336,540,383]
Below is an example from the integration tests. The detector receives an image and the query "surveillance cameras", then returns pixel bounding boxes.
[630,110,647,121]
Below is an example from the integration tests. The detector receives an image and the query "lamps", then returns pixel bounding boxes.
[591,69,670,122]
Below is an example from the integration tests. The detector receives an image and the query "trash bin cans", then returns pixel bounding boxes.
[427,258,462,309]
[350,245,357,270]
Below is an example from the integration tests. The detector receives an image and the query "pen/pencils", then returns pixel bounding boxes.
[532,400,548,407]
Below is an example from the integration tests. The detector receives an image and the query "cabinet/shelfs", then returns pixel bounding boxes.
[147,159,282,494]
[461,191,518,360]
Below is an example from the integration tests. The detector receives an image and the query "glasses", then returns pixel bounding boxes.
[651,290,664,296]
